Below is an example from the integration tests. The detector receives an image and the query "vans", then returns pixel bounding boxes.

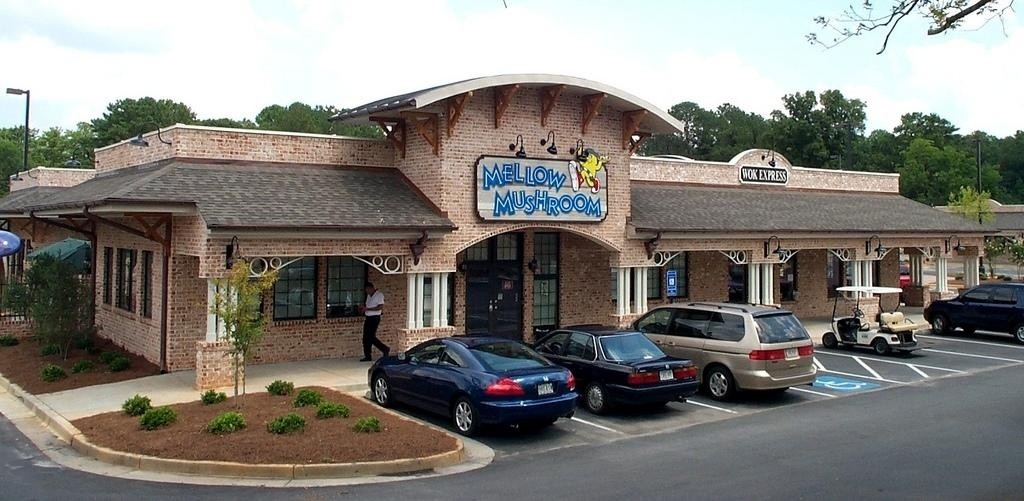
[923,282,1024,345]
[625,300,818,399]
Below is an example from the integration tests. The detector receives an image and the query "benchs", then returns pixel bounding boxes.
[880,313,904,328]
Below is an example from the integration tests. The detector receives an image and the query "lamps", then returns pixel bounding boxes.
[865,235,887,255]
[761,148,778,167]
[764,235,786,259]
[945,235,965,254]
[509,131,557,159]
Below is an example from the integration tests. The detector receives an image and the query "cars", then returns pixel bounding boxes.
[900,262,910,287]
[533,324,700,414]
[367,335,579,437]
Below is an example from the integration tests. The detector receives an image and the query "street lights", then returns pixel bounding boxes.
[7,87,30,170]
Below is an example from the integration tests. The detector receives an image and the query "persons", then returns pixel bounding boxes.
[355,281,390,362]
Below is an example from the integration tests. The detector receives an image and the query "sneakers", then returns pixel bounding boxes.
[383,347,390,357]
[360,357,372,362]
